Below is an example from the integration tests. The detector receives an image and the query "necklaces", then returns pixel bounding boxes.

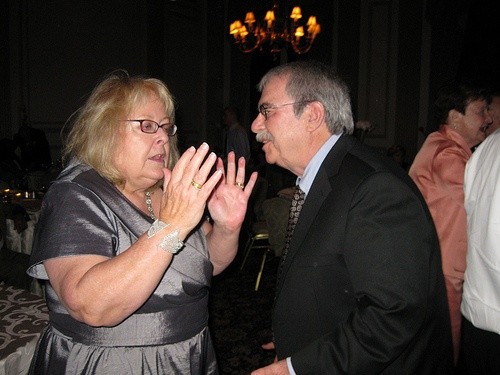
[145,187,156,222]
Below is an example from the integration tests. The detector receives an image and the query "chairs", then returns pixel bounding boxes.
[239,231,291,291]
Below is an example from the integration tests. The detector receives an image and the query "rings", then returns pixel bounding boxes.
[191,181,201,189]
[234,182,244,188]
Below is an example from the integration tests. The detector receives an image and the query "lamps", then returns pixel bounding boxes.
[230,0,320,56]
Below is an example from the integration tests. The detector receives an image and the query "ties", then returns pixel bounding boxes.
[275,186,305,300]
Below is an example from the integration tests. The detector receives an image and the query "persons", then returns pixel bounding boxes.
[387,143,407,166]
[459,128,500,375]
[0,122,51,196]
[470,94,500,152]
[223,107,251,168]
[25,73,258,374]
[408,68,493,374]
[250,64,453,375]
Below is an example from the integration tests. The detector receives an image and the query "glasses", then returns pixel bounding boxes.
[123,119,180,137]
[260,99,316,119]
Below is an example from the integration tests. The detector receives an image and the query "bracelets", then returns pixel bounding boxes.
[147,220,183,255]
